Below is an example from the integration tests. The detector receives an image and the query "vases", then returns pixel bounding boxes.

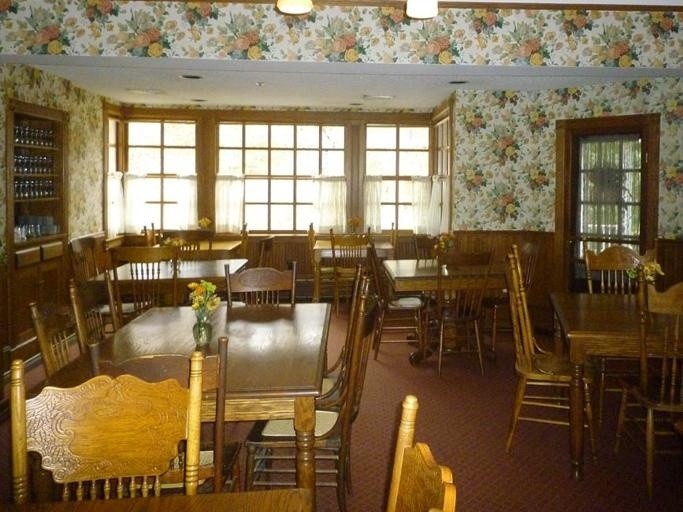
[350,227,355,234]
[193,310,214,347]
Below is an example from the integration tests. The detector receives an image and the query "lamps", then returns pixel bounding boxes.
[405,0,441,22]
[273,0,315,20]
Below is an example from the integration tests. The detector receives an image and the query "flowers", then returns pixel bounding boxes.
[168,2,220,27]
[621,79,653,96]
[553,9,592,40]
[491,128,518,159]
[13,6,70,62]
[491,89,520,105]
[666,161,683,190]
[586,101,618,116]
[522,164,546,190]
[185,277,228,323]
[163,235,185,250]
[491,193,522,221]
[364,8,410,38]
[105,10,175,68]
[592,23,636,69]
[402,19,462,67]
[457,164,490,187]
[521,106,547,135]
[0,0,18,20]
[491,15,552,69]
[468,7,506,41]
[472,57,489,64]
[347,217,364,223]
[307,19,367,63]
[195,217,214,233]
[663,93,682,123]
[436,235,464,255]
[214,19,266,66]
[74,1,124,36]
[457,104,485,137]
[642,8,678,39]
[551,88,588,106]
[625,256,668,280]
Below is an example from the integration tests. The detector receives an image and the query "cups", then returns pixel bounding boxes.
[21,226,26,240]
[13,227,21,242]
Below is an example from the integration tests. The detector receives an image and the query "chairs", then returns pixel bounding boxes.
[510,247,591,357]
[240,284,373,496]
[169,237,216,270]
[143,222,158,252]
[412,235,449,257]
[99,270,127,336]
[389,226,397,252]
[220,258,297,307]
[319,228,367,314]
[64,280,102,350]
[365,236,424,360]
[421,254,487,377]
[26,281,74,375]
[613,263,681,498]
[313,270,370,399]
[250,236,274,277]
[85,335,242,494]
[581,239,659,423]
[72,240,100,284]
[385,395,459,511]
[480,241,537,353]
[121,256,154,311]
[12,346,204,502]
[503,267,588,464]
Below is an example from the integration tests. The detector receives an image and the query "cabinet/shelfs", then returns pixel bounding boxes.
[4,102,75,335]
